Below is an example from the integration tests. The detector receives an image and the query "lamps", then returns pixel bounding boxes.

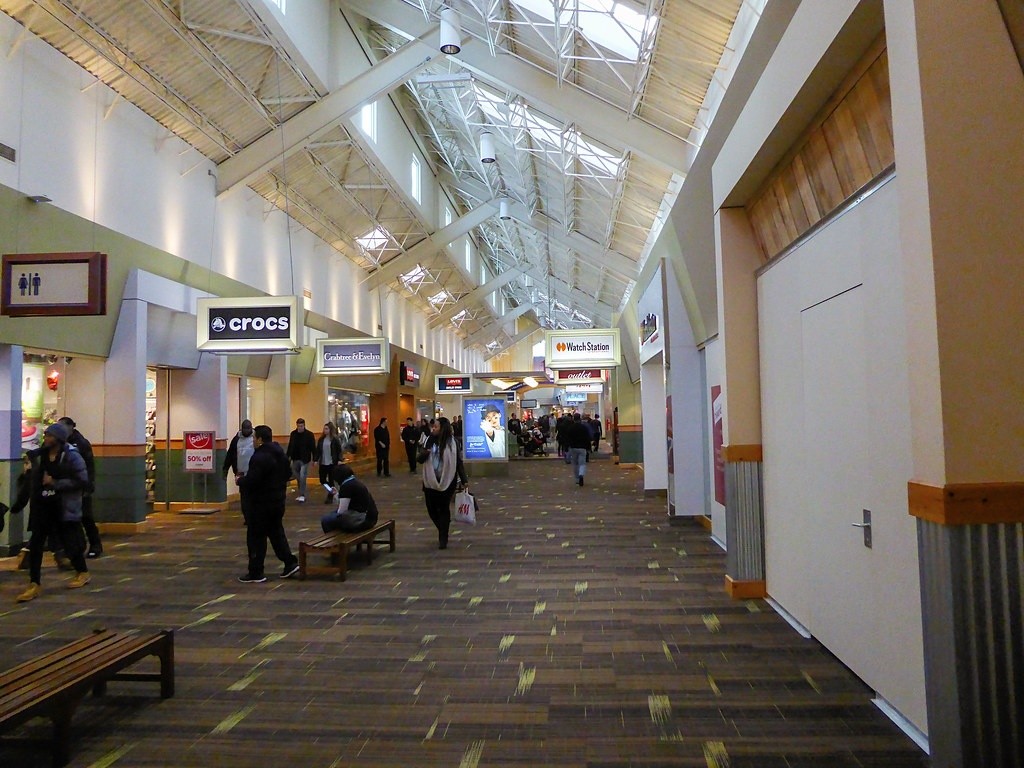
[440,1,461,53]
[526,275,547,328]
[480,130,497,163]
[500,197,511,220]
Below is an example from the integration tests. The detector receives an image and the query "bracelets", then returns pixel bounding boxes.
[314,461,318,464]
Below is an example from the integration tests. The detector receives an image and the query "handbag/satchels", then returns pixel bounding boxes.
[339,508,366,529]
[455,486,478,525]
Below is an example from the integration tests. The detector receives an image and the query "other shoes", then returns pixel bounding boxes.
[410,469,417,474]
[325,493,333,504]
[16,583,41,601]
[385,474,391,477]
[69,572,90,587]
[438,543,446,549]
[576,483,579,486]
[88,543,103,557]
[321,553,331,560]
[376,473,381,477]
[295,496,305,502]
[580,475,584,487]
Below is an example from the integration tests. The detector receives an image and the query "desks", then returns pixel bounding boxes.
[299,519,396,579]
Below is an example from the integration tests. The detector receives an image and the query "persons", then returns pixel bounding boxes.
[0,417,103,601]
[320,464,378,560]
[286,418,316,501]
[417,417,468,549]
[416,418,435,453]
[564,413,592,486]
[313,422,343,504]
[480,405,505,457]
[374,417,391,476]
[222,420,255,492]
[401,417,418,474]
[338,418,361,439]
[451,415,463,451]
[508,409,602,464]
[235,425,299,583]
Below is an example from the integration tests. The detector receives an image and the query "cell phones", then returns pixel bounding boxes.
[323,483,334,494]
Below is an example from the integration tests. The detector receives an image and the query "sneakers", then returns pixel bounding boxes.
[279,562,299,578]
[237,573,267,583]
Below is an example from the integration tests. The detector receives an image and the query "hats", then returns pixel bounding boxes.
[242,420,252,433]
[45,423,69,441]
[481,404,500,420]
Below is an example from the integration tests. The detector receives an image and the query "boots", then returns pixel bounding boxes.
[52,548,71,568]
[14,548,31,569]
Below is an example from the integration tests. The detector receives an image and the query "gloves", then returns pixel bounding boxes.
[480,419,492,433]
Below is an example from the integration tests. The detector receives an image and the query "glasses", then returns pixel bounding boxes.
[42,433,52,437]
[489,417,498,422]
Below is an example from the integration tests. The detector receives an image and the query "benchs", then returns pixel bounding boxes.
[0,624,174,744]
